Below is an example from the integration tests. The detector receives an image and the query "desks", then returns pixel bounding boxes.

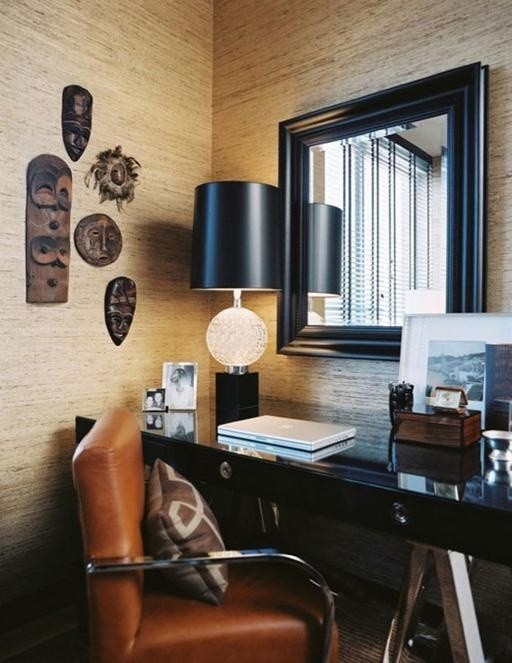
[76,393,512,662]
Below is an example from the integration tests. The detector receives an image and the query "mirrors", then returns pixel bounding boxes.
[275,61,488,364]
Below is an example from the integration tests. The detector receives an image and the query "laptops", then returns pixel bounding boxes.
[217,415,357,451]
[217,436,356,462]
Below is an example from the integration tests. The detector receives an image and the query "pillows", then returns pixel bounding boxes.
[145,459,229,607]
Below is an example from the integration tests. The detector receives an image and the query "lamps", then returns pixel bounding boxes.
[192,181,282,436]
[308,203,343,325]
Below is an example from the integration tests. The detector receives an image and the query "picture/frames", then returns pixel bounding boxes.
[398,474,512,510]
[161,362,197,411]
[398,311,512,430]
[143,388,165,412]
[163,412,199,444]
[145,414,163,430]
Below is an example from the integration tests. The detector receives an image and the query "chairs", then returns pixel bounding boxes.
[73,408,339,662]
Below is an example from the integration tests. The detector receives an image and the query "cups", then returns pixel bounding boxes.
[483,431,512,451]
